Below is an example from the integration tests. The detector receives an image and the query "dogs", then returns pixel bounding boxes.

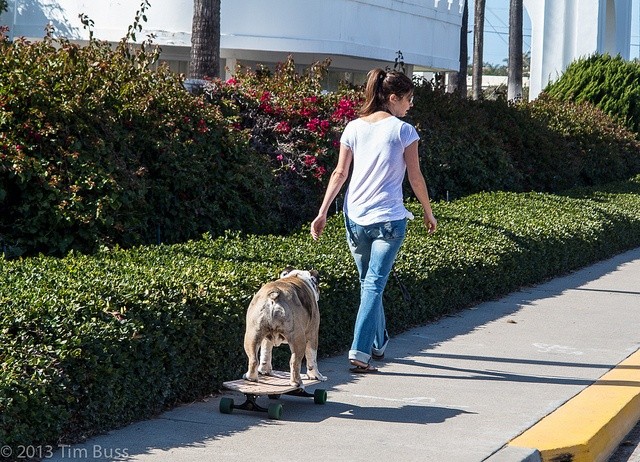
[243,265,327,392]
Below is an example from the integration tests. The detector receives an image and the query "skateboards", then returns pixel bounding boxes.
[220,370,327,419]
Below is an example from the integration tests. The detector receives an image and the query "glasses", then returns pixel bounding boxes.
[404,94,415,103]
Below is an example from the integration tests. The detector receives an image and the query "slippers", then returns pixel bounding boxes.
[349,364,378,373]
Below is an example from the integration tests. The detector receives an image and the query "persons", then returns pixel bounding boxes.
[311,68,440,372]
